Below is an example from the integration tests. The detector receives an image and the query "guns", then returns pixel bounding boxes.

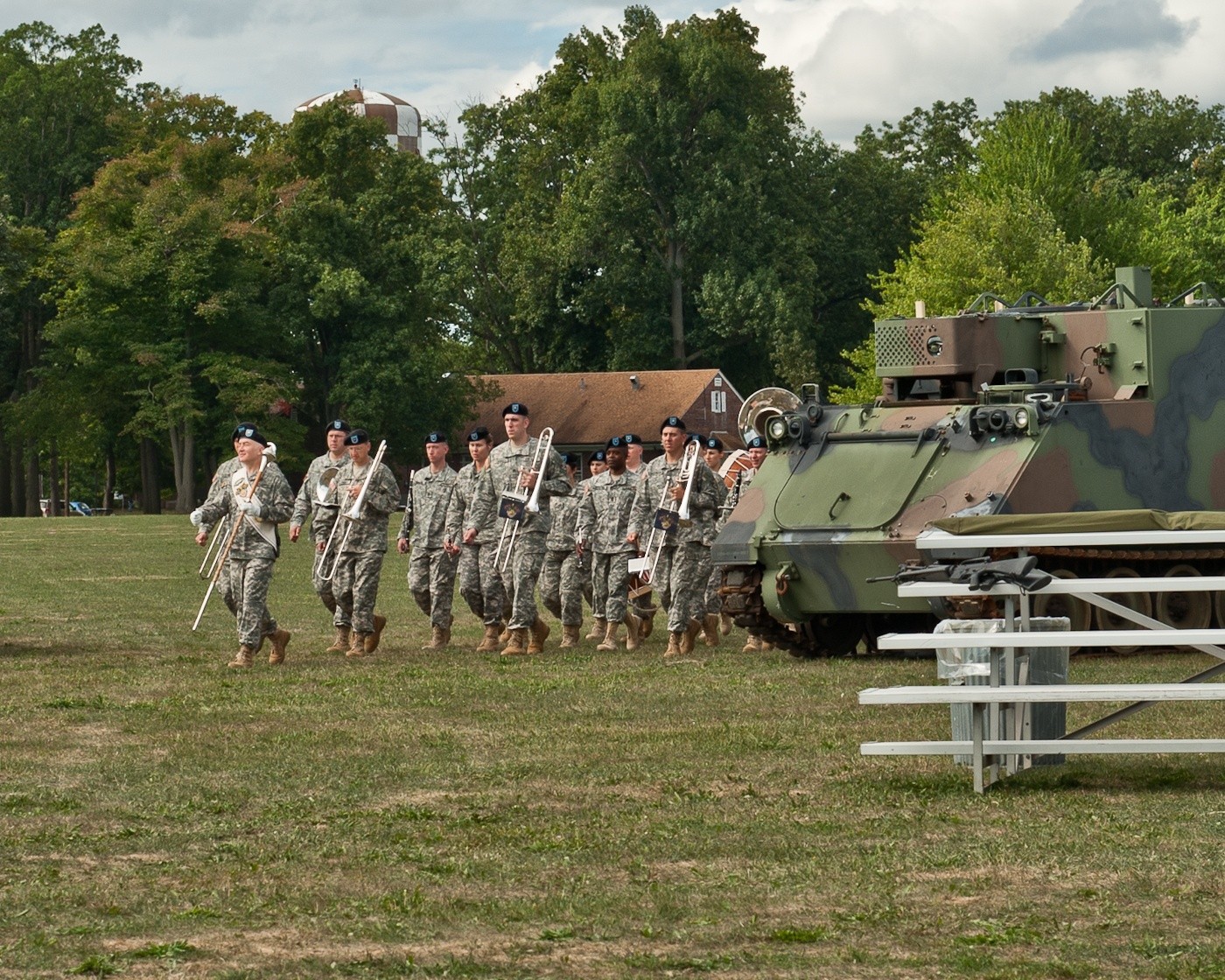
[866,555,1037,591]
[899,563,1052,592]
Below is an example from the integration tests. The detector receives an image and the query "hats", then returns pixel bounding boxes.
[660,417,685,435]
[606,437,627,450]
[622,434,641,445]
[424,431,445,444]
[587,449,605,465]
[502,402,528,417]
[706,438,723,450]
[238,429,266,447]
[747,436,766,451]
[344,429,367,445]
[467,427,489,442]
[325,420,351,436]
[684,434,707,449]
[560,452,577,463]
[232,421,257,439]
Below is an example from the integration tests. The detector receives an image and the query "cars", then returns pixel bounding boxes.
[39,499,91,517]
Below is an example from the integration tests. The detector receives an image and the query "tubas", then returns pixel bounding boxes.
[316,467,341,502]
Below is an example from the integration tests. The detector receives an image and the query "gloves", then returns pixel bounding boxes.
[189,511,202,526]
[240,503,260,516]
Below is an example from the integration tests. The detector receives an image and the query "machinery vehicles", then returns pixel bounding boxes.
[710,267,1225,660]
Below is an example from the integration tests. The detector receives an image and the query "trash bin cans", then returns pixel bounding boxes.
[945,617,1071,768]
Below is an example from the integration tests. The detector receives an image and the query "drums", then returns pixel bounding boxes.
[717,449,754,493]
[627,551,652,599]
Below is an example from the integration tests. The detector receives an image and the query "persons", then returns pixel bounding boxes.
[714,435,767,649]
[288,419,387,653]
[396,403,776,657]
[313,429,401,659]
[575,437,641,651]
[189,422,296,668]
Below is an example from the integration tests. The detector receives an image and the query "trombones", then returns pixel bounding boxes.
[493,427,554,574]
[638,439,700,585]
[199,441,276,581]
[317,440,387,581]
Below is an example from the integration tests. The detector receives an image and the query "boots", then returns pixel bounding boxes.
[476,604,774,657]
[365,615,386,653]
[446,615,453,642]
[423,627,446,650]
[345,633,365,656]
[328,627,352,652]
[268,629,290,666]
[228,646,253,669]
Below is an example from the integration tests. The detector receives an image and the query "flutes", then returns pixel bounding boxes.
[730,469,742,516]
[401,470,414,553]
